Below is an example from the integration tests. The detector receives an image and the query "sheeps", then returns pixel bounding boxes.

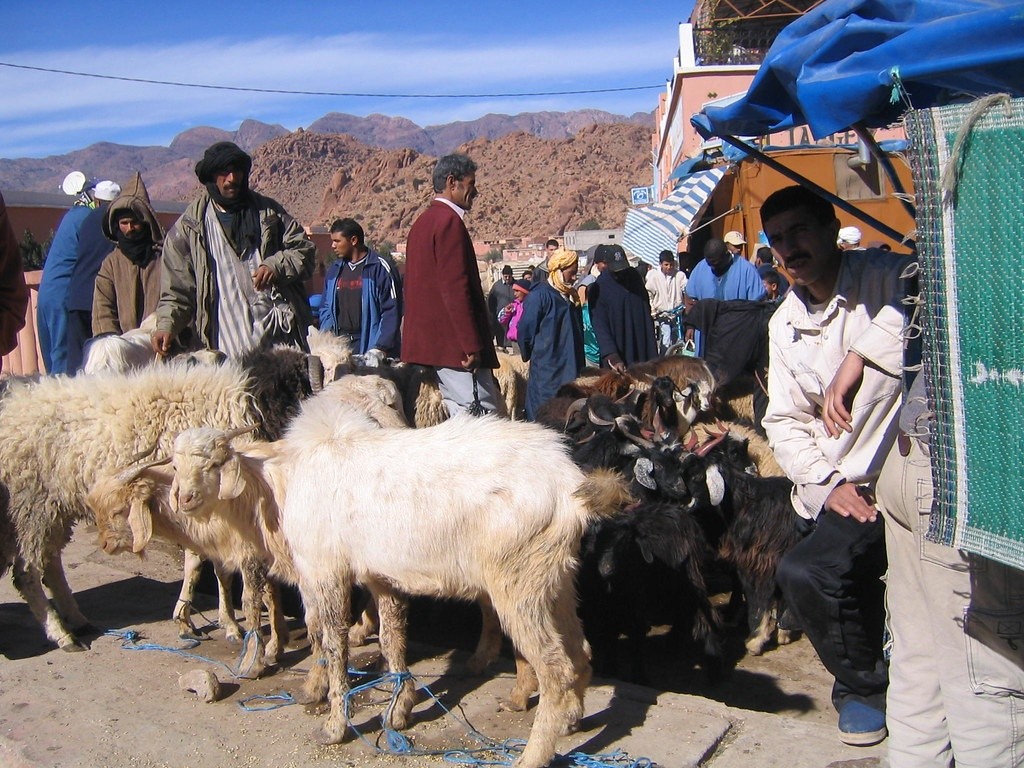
[0,310,818,768]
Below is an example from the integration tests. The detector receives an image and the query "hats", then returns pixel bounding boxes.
[595,244,606,263]
[836,226,862,245]
[605,245,631,273]
[92,180,122,201]
[723,230,747,246]
[512,279,531,294]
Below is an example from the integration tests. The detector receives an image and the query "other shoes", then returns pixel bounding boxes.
[839,687,887,745]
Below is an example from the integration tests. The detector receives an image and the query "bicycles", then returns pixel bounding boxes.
[664,305,686,357]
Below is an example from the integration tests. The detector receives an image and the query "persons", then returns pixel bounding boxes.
[875,365,1024,768]
[150,140,317,366]
[0,190,29,374]
[319,218,402,359]
[399,151,502,421]
[489,225,868,424]
[37,180,124,378]
[91,170,167,339]
[759,184,923,749]
